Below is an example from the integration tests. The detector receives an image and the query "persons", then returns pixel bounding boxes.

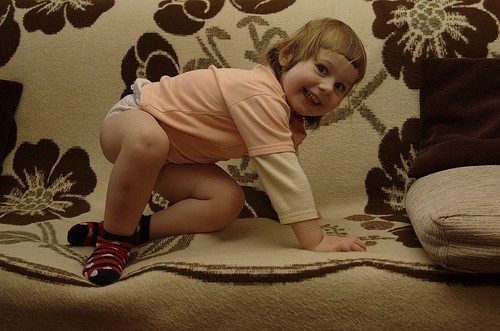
[67,18,368,287]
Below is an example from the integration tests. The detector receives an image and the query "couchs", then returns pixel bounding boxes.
[1,0,500,330]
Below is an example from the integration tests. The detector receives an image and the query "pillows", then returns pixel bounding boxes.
[407,164,500,279]
